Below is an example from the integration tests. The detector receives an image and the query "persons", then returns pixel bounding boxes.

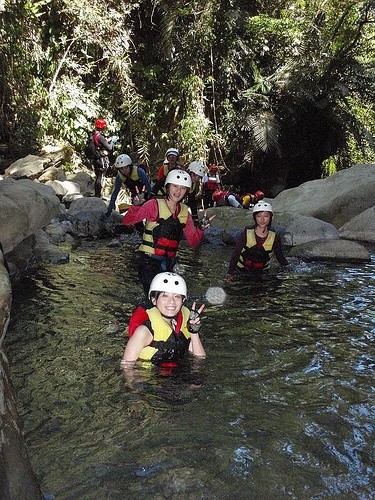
[121,271,206,362]
[121,169,216,300]
[225,201,288,271]
[89,118,116,197]
[105,152,151,233]
[150,147,264,230]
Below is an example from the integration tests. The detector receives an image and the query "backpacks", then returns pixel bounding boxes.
[84,133,104,159]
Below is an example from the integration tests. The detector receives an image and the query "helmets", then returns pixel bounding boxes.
[255,191,264,199]
[148,272,188,301]
[163,169,192,188]
[212,190,224,200]
[95,120,106,128]
[114,154,132,167]
[187,161,205,177]
[253,201,273,213]
[209,166,218,171]
[165,148,179,156]
